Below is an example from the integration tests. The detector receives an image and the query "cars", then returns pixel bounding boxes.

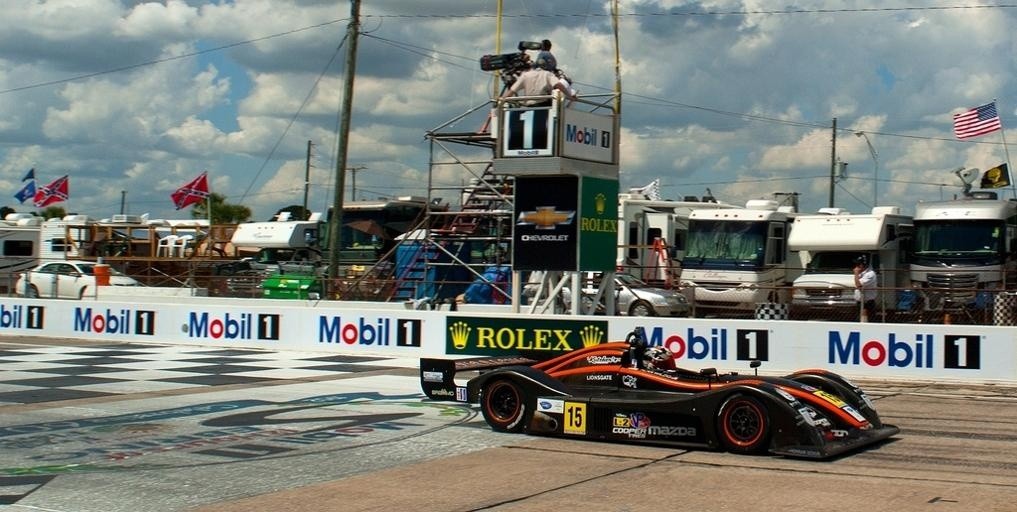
[15,261,143,300]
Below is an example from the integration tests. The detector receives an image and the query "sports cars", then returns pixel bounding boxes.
[420,343,901,461]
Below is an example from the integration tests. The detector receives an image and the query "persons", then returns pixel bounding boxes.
[640,347,677,378]
[537,38,557,71]
[555,70,571,106]
[853,254,878,322]
[503,60,578,148]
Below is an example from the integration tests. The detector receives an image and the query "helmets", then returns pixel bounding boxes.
[643,346,677,374]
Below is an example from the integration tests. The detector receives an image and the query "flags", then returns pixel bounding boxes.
[170,173,207,210]
[13,168,34,203]
[33,174,68,208]
[953,101,1002,139]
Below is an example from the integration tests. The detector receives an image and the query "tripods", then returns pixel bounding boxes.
[479,71,518,133]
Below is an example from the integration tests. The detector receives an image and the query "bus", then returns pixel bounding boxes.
[1,213,208,287]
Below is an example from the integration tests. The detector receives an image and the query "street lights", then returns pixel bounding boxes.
[855,129,879,207]
